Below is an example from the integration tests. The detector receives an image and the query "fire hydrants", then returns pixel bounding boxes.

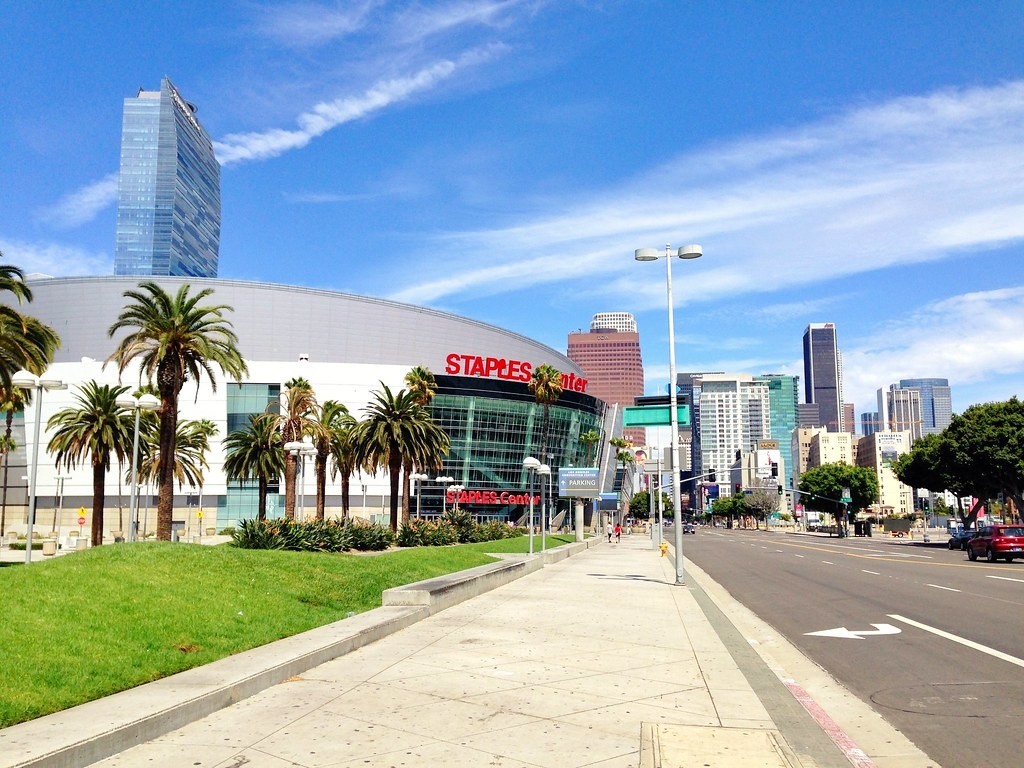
[658,542,668,558]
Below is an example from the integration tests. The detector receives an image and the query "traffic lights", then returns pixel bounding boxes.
[811,491,815,500]
[949,505,954,516]
[736,484,740,493]
[919,499,923,511]
[777,485,783,496]
[846,505,851,514]
[984,501,989,515]
[924,501,929,511]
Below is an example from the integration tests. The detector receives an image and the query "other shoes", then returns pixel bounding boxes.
[609,541,611,543]
[616,542,618,543]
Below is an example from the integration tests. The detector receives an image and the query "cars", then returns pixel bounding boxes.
[948,531,977,551]
[683,525,696,535]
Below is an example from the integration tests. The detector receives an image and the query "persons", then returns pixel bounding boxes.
[607,522,613,542]
[634,450,651,489]
[615,523,622,543]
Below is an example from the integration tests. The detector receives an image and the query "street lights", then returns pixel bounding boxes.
[522,457,541,554]
[447,484,465,515]
[436,476,454,517]
[282,441,318,522]
[53,475,72,552]
[534,464,551,553]
[547,453,554,535]
[409,474,428,520]
[634,241,704,587]
[115,394,161,544]
[10,370,69,565]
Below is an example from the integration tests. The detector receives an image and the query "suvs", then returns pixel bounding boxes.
[967,524,1024,563]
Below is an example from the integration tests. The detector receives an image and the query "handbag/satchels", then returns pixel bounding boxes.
[616,532,618,535]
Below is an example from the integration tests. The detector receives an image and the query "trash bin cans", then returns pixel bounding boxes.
[924,534,930,542]
[854,520,872,537]
